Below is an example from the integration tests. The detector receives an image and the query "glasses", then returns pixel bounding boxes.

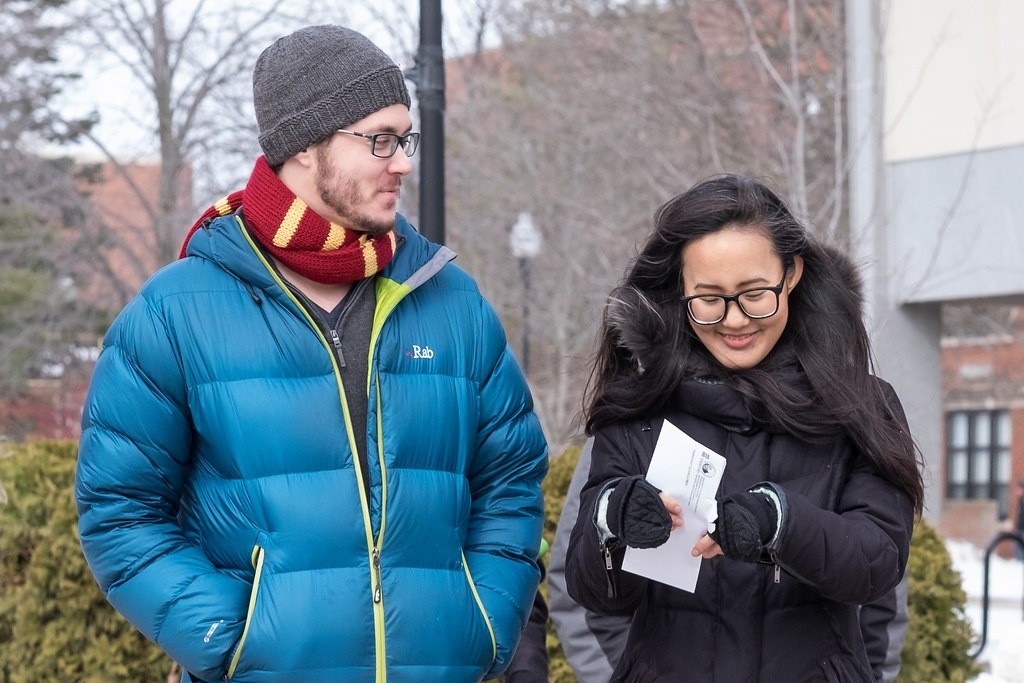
[335,130,421,159]
[678,268,789,326]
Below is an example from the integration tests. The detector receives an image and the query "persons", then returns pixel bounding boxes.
[547,173,923,683]
[57,24,550,683]
[504,538,548,683]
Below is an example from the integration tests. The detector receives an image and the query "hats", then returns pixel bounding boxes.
[252,24,411,166]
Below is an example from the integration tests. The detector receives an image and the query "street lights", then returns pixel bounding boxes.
[507,210,546,374]
